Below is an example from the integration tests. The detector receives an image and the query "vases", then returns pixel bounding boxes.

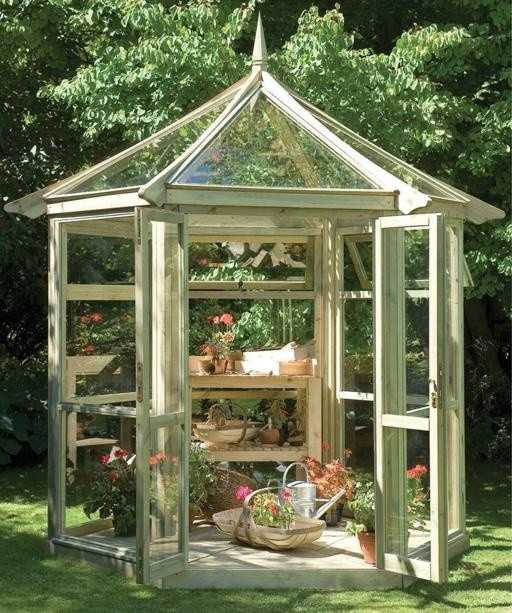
[155,519,176,536]
[210,359,228,374]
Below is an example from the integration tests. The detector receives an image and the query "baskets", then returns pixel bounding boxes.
[194,464,256,522]
[192,421,266,445]
[212,505,326,549]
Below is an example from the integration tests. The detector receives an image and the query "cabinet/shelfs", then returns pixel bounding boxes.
[189,372,321,481]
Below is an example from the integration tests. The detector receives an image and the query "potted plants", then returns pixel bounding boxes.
[259,399,284,444]
[189,449,216,532]
[83,448,136,537]
[305,448,357,527]
[346,482,375,564]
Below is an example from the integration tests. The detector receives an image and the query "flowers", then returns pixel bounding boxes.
[235,486,253,502]
[200,343,218,354]
[84,345,95,355]
[80,313,103,325]
[208,314,235,343]
[148,453,180,466]
[407,465,428,479]
[267,487,293,521]
